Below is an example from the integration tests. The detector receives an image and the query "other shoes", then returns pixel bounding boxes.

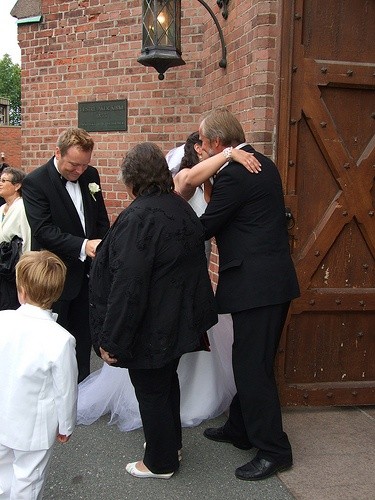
[143,441,182,461]
[126,461,173,479]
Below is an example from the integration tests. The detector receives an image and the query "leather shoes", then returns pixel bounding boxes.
[204,427,251,450]
[235,452,291,481]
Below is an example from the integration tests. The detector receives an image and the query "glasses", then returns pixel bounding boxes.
[0,178,13,183]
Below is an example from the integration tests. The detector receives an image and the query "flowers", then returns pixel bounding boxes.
[88,183,101,202]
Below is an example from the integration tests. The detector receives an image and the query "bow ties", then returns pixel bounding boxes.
[62,176,78,186]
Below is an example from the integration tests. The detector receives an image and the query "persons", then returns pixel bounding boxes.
[21,126,110,384]
[0,162,31,310]
[77,131,261,432]
[199,106,301,481]
[89,142,218,479]
[0,249,78,500]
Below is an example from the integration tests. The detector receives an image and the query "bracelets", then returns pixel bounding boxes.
[223,146,232,163]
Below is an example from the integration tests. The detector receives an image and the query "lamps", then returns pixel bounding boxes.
[136,0,229,81]
